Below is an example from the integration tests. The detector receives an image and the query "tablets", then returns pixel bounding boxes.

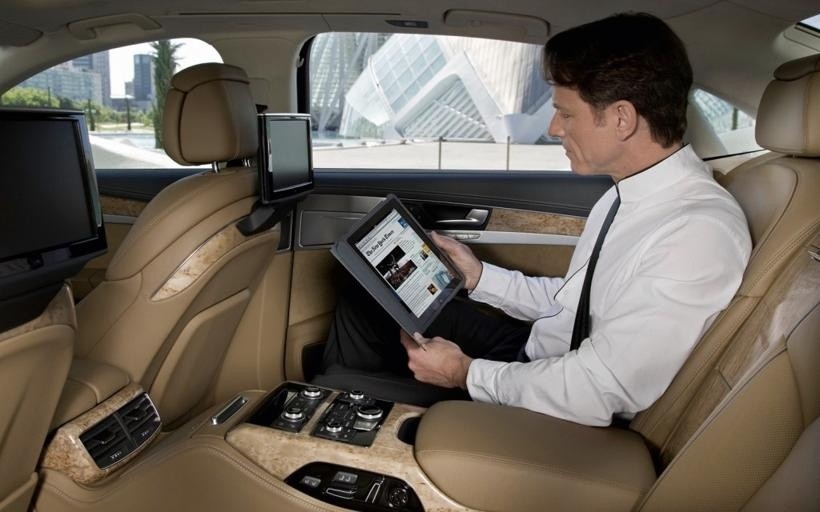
[342,193,466,328]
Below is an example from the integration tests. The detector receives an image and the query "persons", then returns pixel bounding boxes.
[323,11,752,429]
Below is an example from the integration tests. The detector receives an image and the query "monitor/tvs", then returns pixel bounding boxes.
[0,107,108,265]
[237,113,316,206]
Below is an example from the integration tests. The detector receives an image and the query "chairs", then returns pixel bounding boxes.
[0,282,77,512]
[306,50,819,454]
[77,61,282,430]
[636,303,818,512]
